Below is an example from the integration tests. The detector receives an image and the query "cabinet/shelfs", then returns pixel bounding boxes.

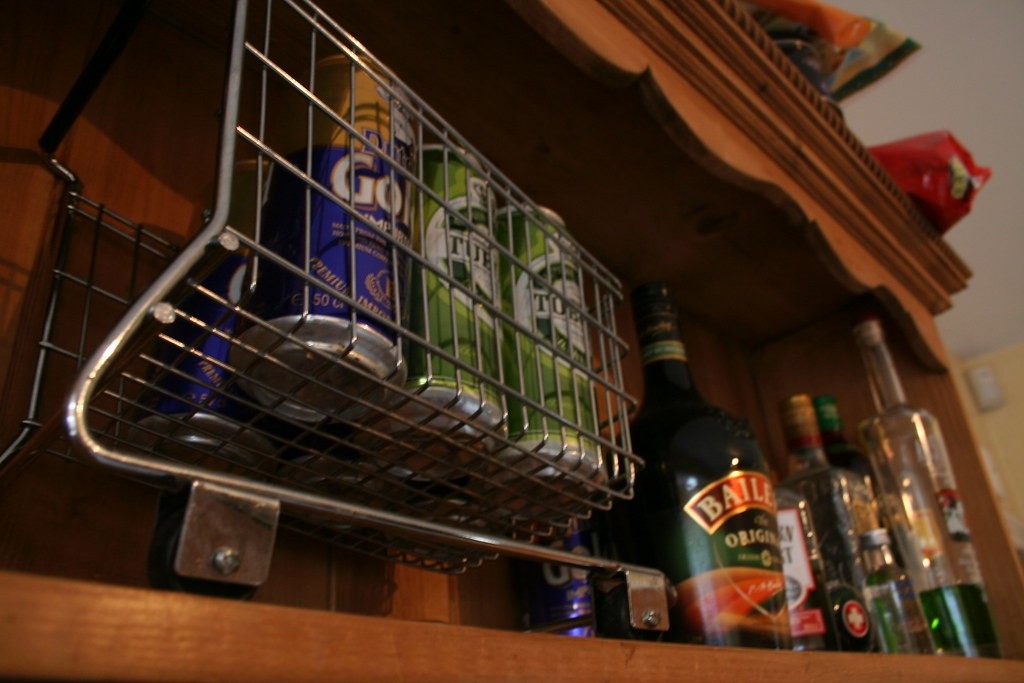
[1,0,1024,683]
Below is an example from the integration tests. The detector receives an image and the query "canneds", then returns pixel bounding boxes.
[506,523,596,637]
[128,53,607,558]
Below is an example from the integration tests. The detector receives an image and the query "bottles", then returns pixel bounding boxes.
[851,316,1000,660]
[756,464,837,654]
[606,270,793,651]
[858,527,937,656]
[814,391,905,577]
[777,393,893,656]
